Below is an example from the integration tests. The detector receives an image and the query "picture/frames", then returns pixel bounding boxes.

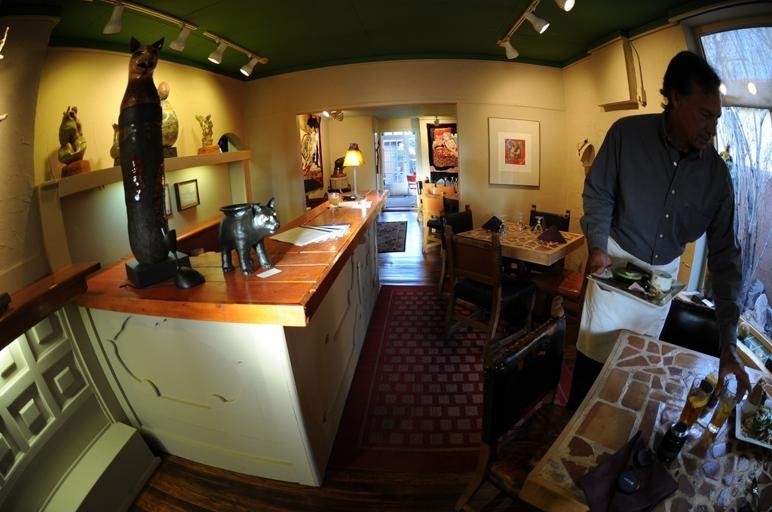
[173,178,203,213]
[487,115,542,189]
[426,122,457,184]
[298,112,324,190]
[160,182,173,218]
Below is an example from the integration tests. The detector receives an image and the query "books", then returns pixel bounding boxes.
[270,222,350,247]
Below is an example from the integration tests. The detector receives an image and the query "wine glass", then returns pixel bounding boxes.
[499,214,543,239]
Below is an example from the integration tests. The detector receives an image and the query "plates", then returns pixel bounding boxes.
[614,268,642,280]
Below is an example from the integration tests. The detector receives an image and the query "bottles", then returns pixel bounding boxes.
[742,378,767,416]
[661,421,688,459]
[444,176,458,194]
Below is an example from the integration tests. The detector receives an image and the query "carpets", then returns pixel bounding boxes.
[377,218,409,255]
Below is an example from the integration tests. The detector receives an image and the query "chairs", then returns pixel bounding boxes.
[418,177,459,254]
[449,294,579,512]
[440,204,595,376]
[657,297,722,359]
[406,172,417,193]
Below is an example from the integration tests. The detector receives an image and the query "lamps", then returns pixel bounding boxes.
[202,28,271,79]
[321,107,344,123]
[431,113,442,125]
[343,143,364,200]
[102,0,201,61]
[494,0,578,65]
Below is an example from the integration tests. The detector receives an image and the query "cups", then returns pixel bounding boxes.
[650,270,672,292]
[680,376,714,427]
[328,192,339,211]
[709,379,744,434]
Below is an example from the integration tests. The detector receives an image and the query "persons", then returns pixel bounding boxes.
[567,50,752,414]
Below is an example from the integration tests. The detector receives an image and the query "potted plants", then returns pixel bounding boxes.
[194,112,220,154]
